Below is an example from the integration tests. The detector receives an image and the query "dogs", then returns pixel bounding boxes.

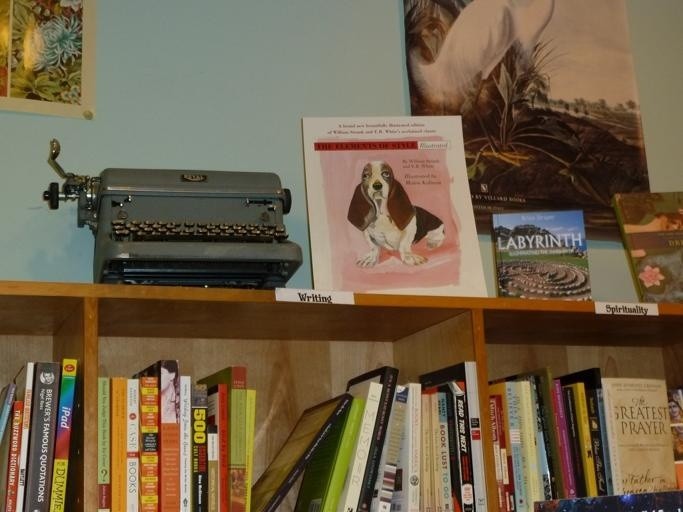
[346,159,447,269]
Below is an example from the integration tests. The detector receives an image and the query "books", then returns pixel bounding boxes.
[492,209,593,300]
[301,115,488,297]
[612,192,683,303]
[0,359,256,511]
[251,362,683,512]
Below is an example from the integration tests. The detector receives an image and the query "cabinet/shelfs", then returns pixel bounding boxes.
[0,280,683,512]
